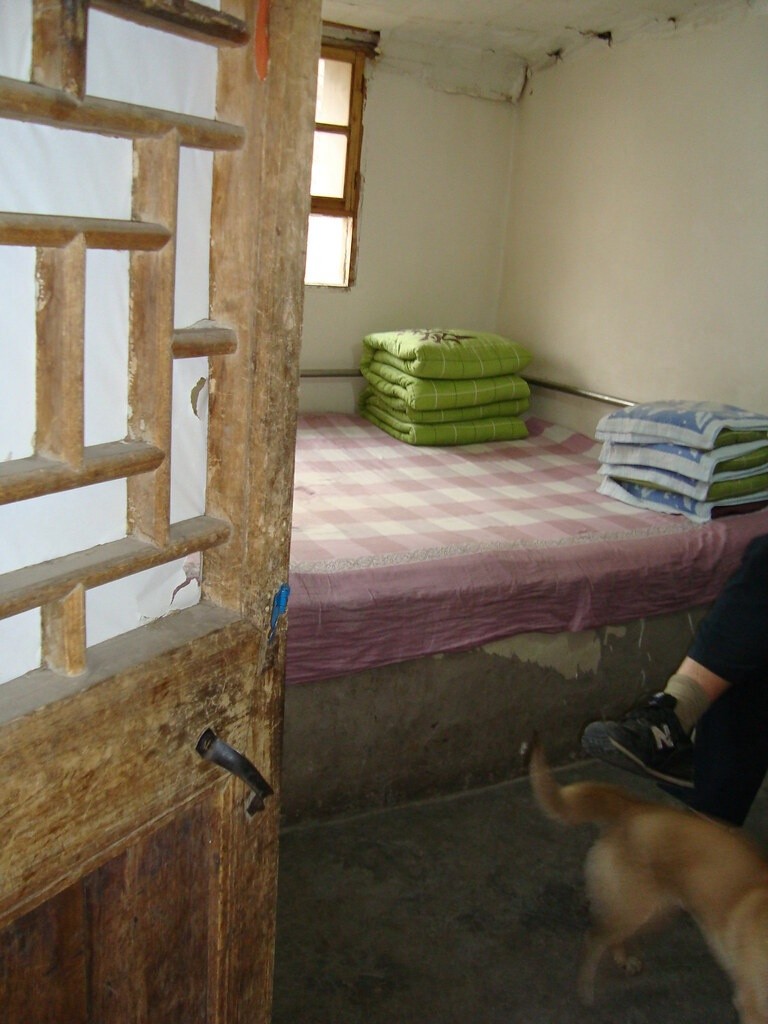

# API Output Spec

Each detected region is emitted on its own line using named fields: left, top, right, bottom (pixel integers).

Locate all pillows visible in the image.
left=594, top=399, right=767, bottom=525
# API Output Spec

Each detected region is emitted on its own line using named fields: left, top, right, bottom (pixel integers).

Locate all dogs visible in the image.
left=530, top=735, right=768, bottom=1024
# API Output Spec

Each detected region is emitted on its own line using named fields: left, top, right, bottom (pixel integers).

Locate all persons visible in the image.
left=579, top=531, right=768, bottom=833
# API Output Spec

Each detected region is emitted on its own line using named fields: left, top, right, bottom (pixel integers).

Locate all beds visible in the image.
left=283, top=408, right=767, bottom=690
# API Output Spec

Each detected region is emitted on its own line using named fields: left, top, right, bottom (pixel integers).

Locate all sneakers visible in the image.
left=582, top=692, right=704, bottom=791
